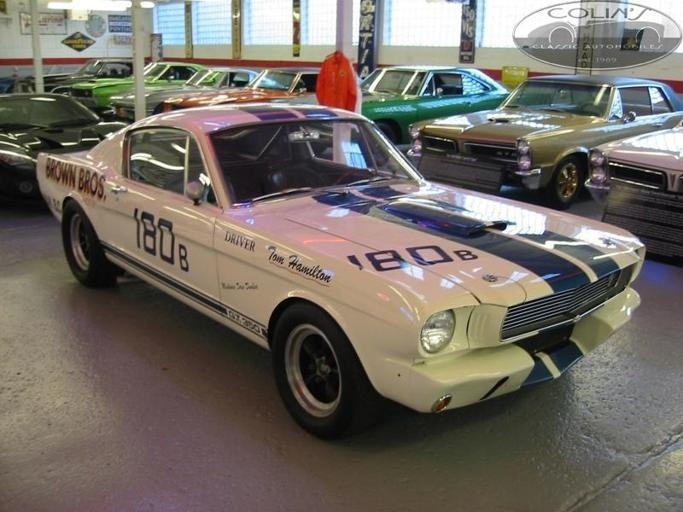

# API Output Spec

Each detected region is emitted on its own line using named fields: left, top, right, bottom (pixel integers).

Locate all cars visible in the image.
left=33, top=99, right=650, bottom=439
